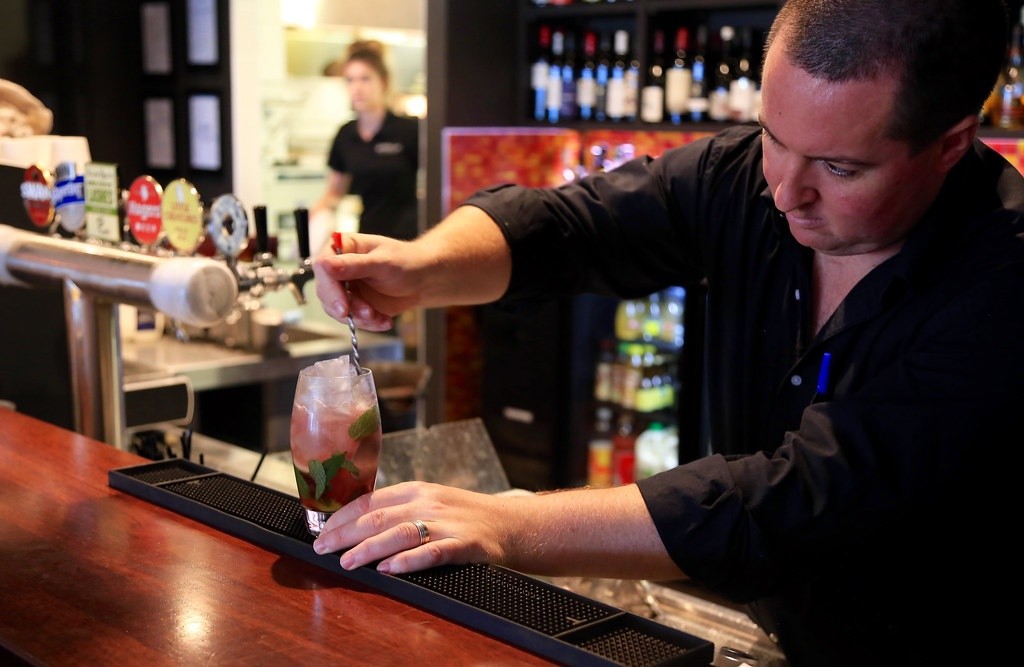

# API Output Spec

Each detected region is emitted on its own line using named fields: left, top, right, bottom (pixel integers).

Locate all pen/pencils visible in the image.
left=816, top=352, right=831, bottom=394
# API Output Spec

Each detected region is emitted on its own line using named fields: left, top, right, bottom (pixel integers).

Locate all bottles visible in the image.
left=990, top=51, right=1024, bottom=132
left=527, top=23, right=764, bottom=186
left=587, top=286, right=685, bottom=489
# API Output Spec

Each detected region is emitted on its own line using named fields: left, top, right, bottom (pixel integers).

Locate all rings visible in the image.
left=412, top=520, right=430, bottom=544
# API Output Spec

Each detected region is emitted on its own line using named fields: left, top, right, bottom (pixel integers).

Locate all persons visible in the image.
left=313, top=0, right=1024, bottom=667
left=310, top=42, right=419, bottom=242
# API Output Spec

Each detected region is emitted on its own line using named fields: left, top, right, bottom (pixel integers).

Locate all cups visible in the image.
left=289, top=364, right=383, bottom=538
